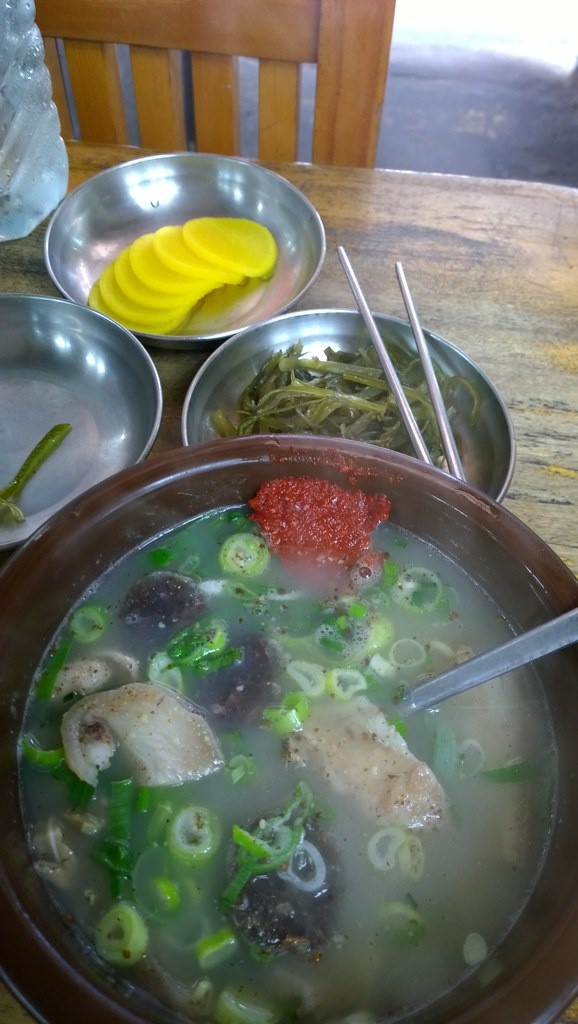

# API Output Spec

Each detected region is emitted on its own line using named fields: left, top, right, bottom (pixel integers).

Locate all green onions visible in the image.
left=19, top=508, right=533, bottom=1024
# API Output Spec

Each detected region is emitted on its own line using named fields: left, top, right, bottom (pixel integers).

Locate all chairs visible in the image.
left=33, top=0, right=396, bottom=169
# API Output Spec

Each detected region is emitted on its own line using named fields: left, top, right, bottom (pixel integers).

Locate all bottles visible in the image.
left=0, top=0, right=69, bottom=241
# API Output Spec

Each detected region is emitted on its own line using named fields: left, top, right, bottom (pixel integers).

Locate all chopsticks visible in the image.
left=335, top=247, right=464, bottom=482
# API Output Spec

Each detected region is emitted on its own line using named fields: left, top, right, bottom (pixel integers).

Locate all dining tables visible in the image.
left=2, top=141, right=577, bottom=579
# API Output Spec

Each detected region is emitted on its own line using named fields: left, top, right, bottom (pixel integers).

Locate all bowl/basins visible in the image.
left=180, top=307, right=517, bottom=506
left=1, top=433, right=578, bottom=1023
left=43, top=152, right=327, bottom=347
left=0, top=291, right=163, bottom=548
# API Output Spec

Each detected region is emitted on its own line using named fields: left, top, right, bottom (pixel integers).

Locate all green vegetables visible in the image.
left=207, top=342, right=481, bottom=474
left=0, top=423, right=72, bottom=520
